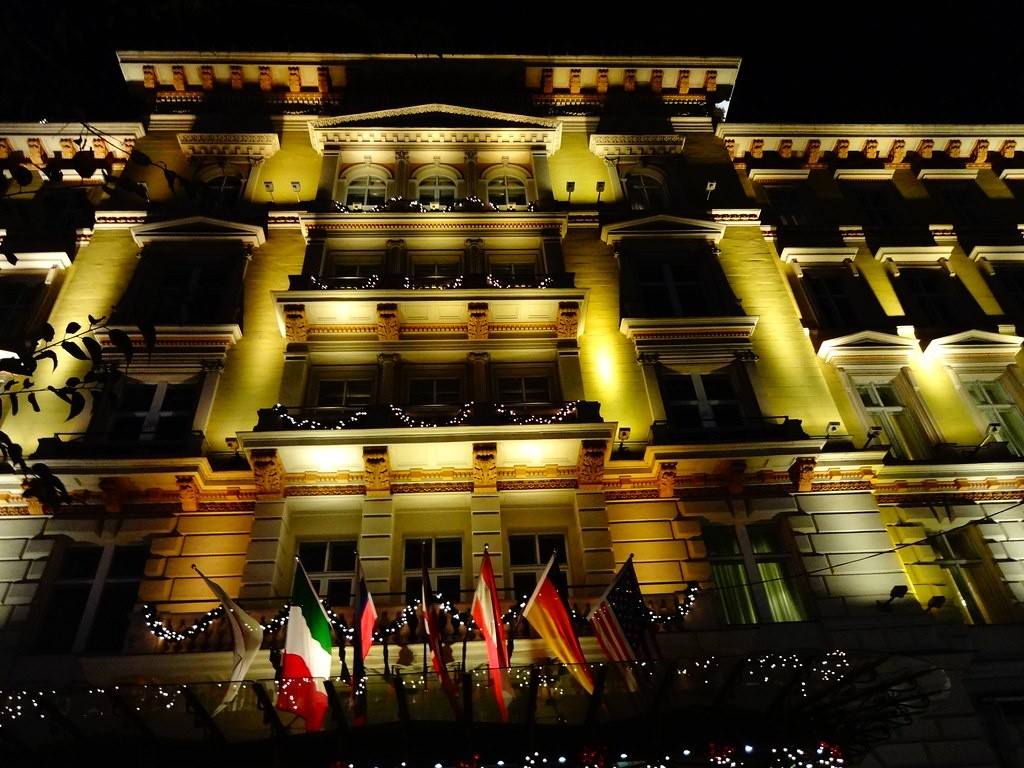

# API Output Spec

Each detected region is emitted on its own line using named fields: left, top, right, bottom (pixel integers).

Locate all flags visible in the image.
left=469, top=549, right=508, bottom=725
left=422, top=545, right=456, bottom=707
left=587, top=557, right=666, bottom=692
left=352, top=557, right=378, bottom=729
left=522, top=554, right=594, bottom=697
left=276, top=560, right=332, bottom=733
left=204, top=578, right=264, bottom=718
